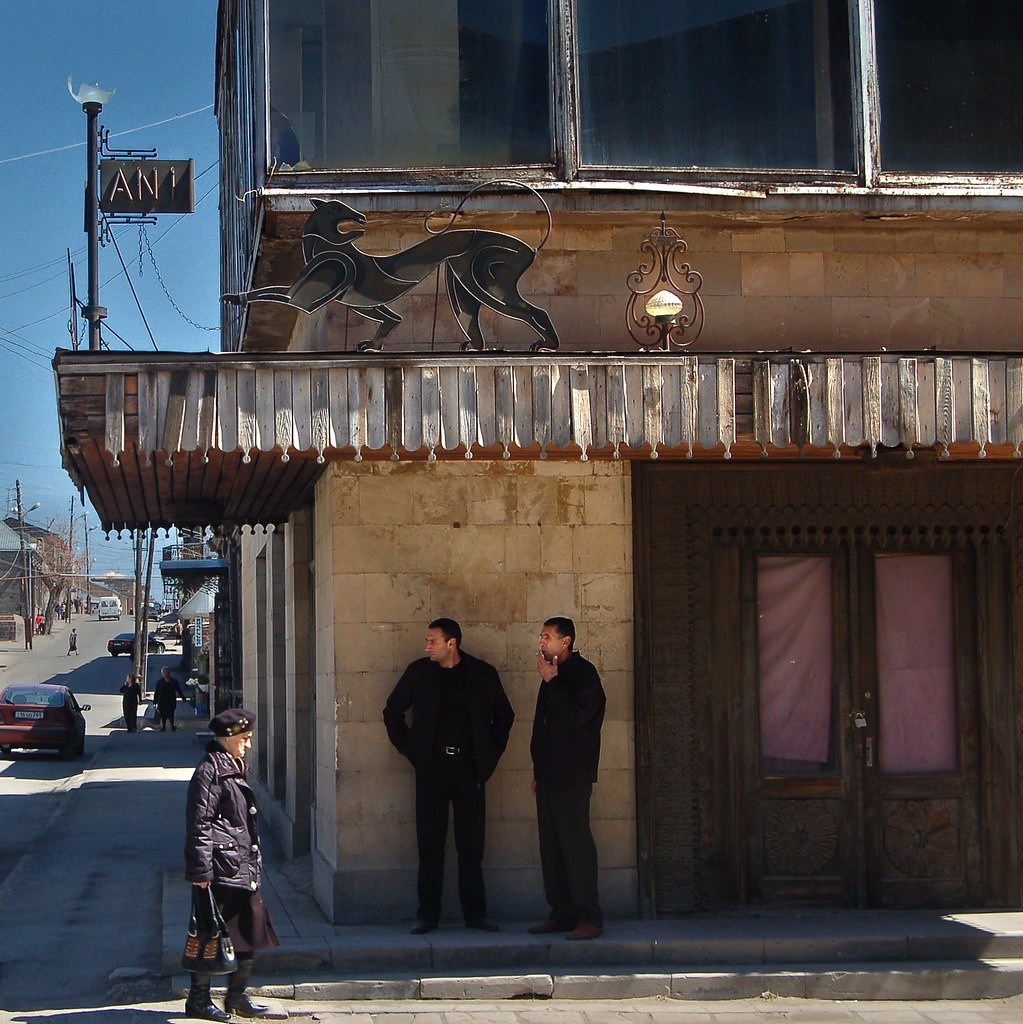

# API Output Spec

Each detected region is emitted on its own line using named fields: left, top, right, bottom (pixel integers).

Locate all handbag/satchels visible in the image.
left=143, top=703, right=161, bottom=725
left=181, top=886, right=238, bottom=975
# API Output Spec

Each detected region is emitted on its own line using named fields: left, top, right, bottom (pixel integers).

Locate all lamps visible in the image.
left=623, top=211, right=707, bottom=356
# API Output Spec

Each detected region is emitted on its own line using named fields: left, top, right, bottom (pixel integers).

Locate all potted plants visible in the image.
left=194, top=654, right=207, bottom=704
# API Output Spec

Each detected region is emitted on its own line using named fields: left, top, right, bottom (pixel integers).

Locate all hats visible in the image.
left=208, top=708, right=256, bottom=737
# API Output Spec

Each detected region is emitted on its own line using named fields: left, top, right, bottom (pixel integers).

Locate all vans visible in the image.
left=98, top=596, right=123, bottom=621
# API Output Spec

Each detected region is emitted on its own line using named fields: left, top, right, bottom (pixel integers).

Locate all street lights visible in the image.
left=65, top=511, right=90, bottom=624
left=85, top=524, right=100, bottom=614
left=18, top=503, right=40, bottom=650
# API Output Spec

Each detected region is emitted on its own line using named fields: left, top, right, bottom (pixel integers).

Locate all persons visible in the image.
left=55, top=602, right=66, bottom=620
left=67, top=628, right=80, bottom=656
left=382, top=618, right=516, bottom=934
left=61, top=599, right=66, bottom=605
left=174, top=619, right=183, bottom=646
left=153, top=666, right=186, bottom=732
left=119, top=673, right=142, bottom=733
left=70, top=600, right=73, bottom=614
left=74, top=596, right=82, bottom=613
left=102, top=601, right=106, bottom=607
left=36, top=613, right=45, bottom=635
left=112, top=602, right=115, bottom=606
left=529, top=616, right=607, bottom=942
left=185, top=708, right=280, bottom=1021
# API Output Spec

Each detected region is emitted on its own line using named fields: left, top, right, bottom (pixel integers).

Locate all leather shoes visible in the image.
left=529, top=920, right=574, bottom=932
left=566, top=923, right=602, bottom=939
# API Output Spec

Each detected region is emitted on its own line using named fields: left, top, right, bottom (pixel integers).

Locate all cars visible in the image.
left=107, top=632, right=165, bottom=657
left=141, top=607, right=158, bottom=622
left=0, top=684, right=91, bottom=760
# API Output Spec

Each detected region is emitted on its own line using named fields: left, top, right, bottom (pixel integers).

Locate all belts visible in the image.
left=434, top=745, right=471, bottom=755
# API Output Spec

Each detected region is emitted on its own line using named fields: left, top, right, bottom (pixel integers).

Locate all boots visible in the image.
left=185, top=972, right=231, bottom=1021
left=225, top=958, right=268, bottom=1013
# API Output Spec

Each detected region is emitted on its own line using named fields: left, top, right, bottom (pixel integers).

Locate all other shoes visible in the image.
left=67, top=654, right=71, bottom=656
left=160, top=727, right=164, bottom=731
left=76, top=653, right=79, bottom=655
left=411, top=920, right=439, bottom=934
left=172, top=726, right=177, bottom=731
left=466, top=919, right=497, bottom=931
